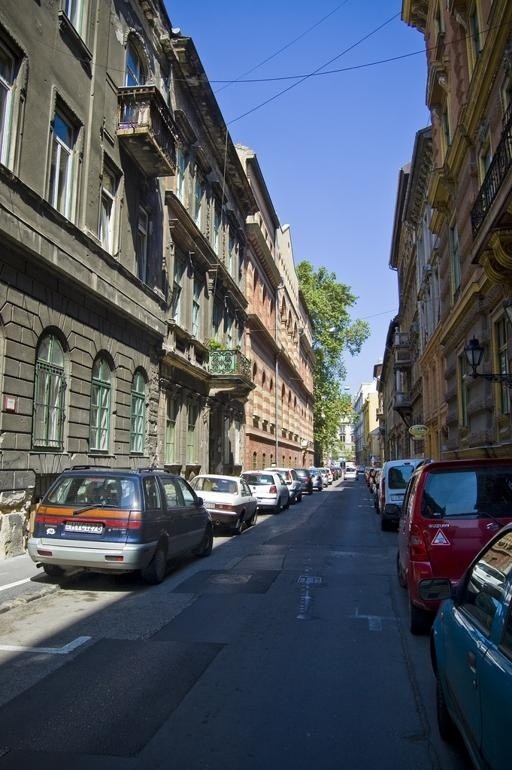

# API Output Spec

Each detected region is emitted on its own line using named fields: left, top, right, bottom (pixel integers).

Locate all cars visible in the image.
left=343, top=465, right=359, bottom=481
left=294, top=466, right=342, bottom=494
left=427, top=521, right=511, bottom=770
left=394, top=457, right=511, bottom=634
left=239, top=470, right=289, bottom=514
left=181, top=474, right=258, bottom=535
left=266, top=466, right=302, bottom=504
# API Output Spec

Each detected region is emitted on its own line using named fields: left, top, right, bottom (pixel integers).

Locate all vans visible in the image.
left=376, top=458, right=434, bottom=531
left=27, top=464, right=214, bottom=583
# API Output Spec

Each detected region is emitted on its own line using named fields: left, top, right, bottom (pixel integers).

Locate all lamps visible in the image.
left=462, top=335, right=511, bottom=390
